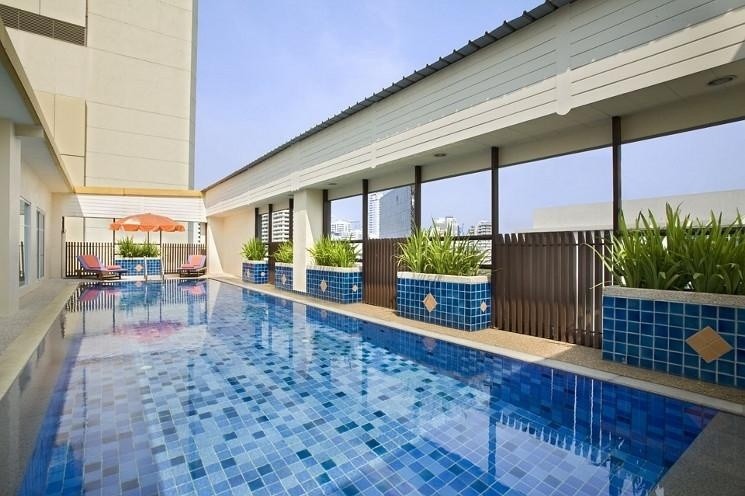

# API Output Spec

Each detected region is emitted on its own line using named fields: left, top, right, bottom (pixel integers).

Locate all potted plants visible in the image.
left=306, top=231, right=366, bottom=306
left=114, top=234, right=163, bottom=277
left=271, top=237, right=294, bottom=292
left=389, top=214, right=492, bottom=332
left=239, top=236, right=269, bottom=285
left=576, top=201, right=745, bottom=392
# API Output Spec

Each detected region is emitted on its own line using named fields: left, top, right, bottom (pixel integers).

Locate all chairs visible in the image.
left=177, top=279, right=208, bottom=297
left=175, top=254, right=207, bottom=278
left=77, top=284, right=128, bottom=303
left=75, top=254, right=127, bottom=282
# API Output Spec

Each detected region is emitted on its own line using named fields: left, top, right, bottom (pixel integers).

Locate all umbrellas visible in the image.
left=113, top=307, right=185, bottom=345
left=109, top=213, right=185, bottom=247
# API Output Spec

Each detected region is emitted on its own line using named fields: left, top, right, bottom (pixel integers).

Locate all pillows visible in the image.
left=181, top=263, right=195, bottom=269
left=105, top=264, right=122, bottom=271
